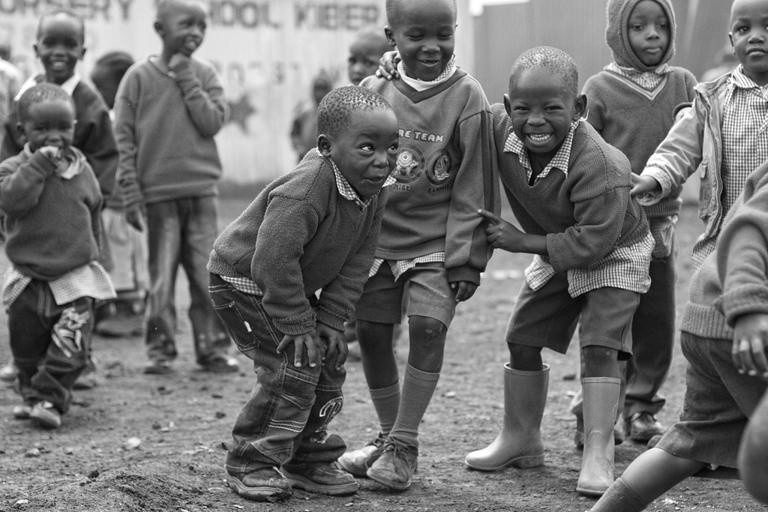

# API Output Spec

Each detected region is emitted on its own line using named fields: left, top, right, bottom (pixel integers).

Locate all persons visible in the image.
left=572, top=0, right=701, bottom=449
left=206, top=85, right=400, bottom=502
left=339, top=0, right=502, bottom=489
left=1, top=83, right=104, bottom=428
left=627, top=0, right=767, bottom=262
left=1, top=12, right=120, bottom=204
left=342, top=27, right=402, bottom=361
left=376, top=50, right=656, bottom=497
left=588, top=160, right=768, bottom=512
left=90, top=50, right=154, bottom=307
left=290, top=75, right=334, bottom=164
left=111, top=0, right=242, bottom=377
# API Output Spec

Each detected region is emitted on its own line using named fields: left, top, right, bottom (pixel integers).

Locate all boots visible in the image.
left=465, top=363, right=550, bottom=471
left=576, top=377, right=620, bottom=495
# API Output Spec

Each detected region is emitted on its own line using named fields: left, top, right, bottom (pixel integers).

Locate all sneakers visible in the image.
left=73, top=360, right=96, bottom=388
left=573, top=416, right=622, bottom=445
left=196, top=350, right=238, bottom=371
left=146, top=358, right=169, bottom=373
left=337, top=431, right=385, bottom=477
left=622, top=410, right=665, bottom=441
left=280, top=461, right=358, bottom=495
left=366, top=437, right=419, bottom=490
left=95, top=300, right=144, bottom=334
left=30, top=400, right=61, bottom=426
left=225, top=467, right=289, bottom=499
left=13, top=400, right=32, bottom=416
